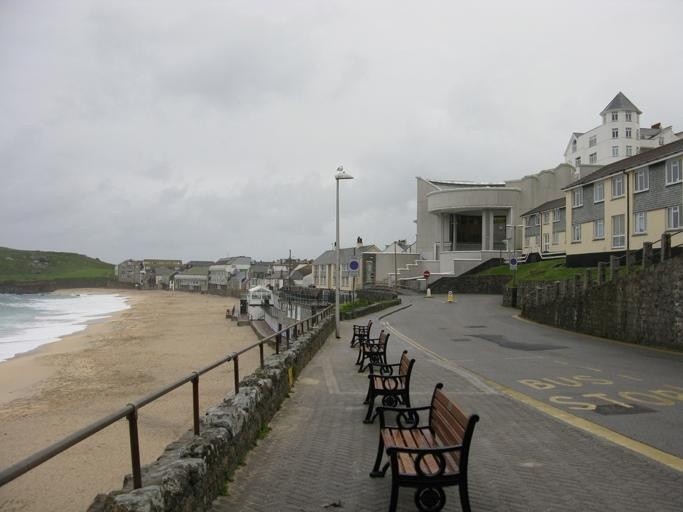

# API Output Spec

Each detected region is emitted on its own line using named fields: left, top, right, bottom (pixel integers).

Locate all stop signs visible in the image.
left=423, top=271, right=429, bottom=279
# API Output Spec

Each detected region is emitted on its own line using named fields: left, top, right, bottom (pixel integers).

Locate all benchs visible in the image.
left=367, top=381, right=479, bottom=512
left=350, top=319, right=373, bottom=347
left=362, top=348, right=416, bottom=421
left=354, top=328, right=389, bottom=373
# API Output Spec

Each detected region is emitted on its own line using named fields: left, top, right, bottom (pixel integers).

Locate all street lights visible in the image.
left=384, top=239, right=407, bottom=294
left=506, top=224, right=524, bottom=252
left=332, top=164, right=353, bottom=338
